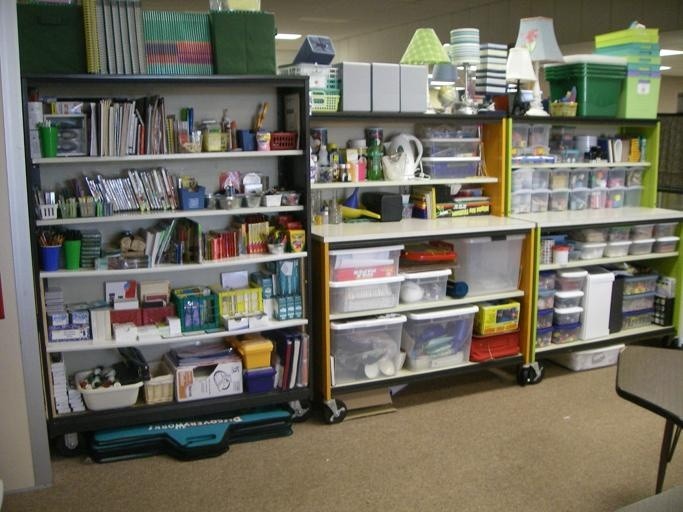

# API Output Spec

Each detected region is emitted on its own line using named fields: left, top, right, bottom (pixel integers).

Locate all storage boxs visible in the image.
left=165, top=334, right=276, bottom=404
left=544, top=27, right=663, bottom=120
left=333, top=62, right=429, bottom=113
left=72, top=366, right=143, bottom=411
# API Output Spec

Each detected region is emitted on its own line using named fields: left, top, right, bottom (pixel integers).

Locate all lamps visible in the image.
left=474, top=41, right=509, bottom=97
left=447, top=27, right=480, bottom=113
left=431, top=43, right=461, bottom=114
left=513, top=15, right=564, bottom=117
left=400, top=27, right=449, bottom=65
left=503, top=48, right=534, bottom=116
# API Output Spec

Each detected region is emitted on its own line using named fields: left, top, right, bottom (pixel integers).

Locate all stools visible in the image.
left=618, top=342, right=682, bottom=495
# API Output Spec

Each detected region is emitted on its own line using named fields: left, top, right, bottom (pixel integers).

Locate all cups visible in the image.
left=39, top=245, right=62, bottom=272
left=38, top=127, right=58, bottom=159
left=540, top=236, right=554, bottom=263
left=64, top=240, right=81, bottom=271
left=176, top=135, right=202, bottom=153
left=401, top=203, right=414, bottom=222
left=266, top=243, right=284, bottom=254
left=381, top=152, right=406, bottom=181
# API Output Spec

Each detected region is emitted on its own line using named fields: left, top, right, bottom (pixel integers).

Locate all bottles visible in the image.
left=575, top=133, right=648, bottom=163
left=310, top=126, right=382, bottom=184
left=199, top=118, right=270, bottom=152
left=80, top=365, right=121, bottom=390
left=447, top=279, right=468, bottom=298
left=279, top=187, right=300, bottom=205
left=552, top=246, right=570, bottom=264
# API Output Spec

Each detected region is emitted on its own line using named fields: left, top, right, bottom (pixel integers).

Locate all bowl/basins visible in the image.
left=204, top=194, right=261, bottom=209
left=310, top=191, right=343, bottom=226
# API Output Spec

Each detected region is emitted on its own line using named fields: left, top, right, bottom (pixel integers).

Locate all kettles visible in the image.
left=382, top=133, right=427, bottom=182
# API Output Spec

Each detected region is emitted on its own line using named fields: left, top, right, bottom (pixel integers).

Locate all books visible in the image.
left=66, top=95, right=167, bottom=157
left=76, top=166, right=176, bottom=213
left=83, top=1, right=216, bottom=78
left=475, top=41, right=509, bottom=98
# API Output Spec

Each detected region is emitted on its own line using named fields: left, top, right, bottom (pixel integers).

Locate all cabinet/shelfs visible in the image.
left=19, top=70, right=313, bottom=459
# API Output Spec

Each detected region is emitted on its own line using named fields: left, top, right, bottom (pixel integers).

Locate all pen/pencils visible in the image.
left=38, top=231, right=65, bottom=247
left=60, top=228, right=83, bottom=240
left=255, top=102, right=269, bottom=129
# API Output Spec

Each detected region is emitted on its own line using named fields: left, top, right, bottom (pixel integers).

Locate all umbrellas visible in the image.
left=144, top=212, right=269, bottom=265
